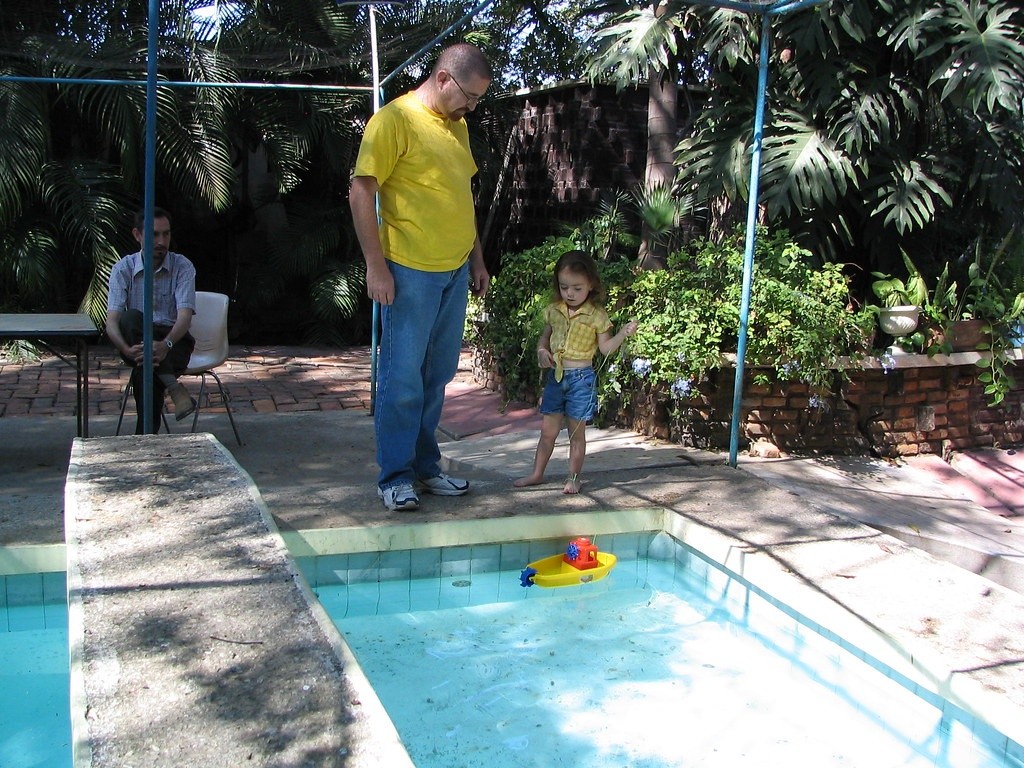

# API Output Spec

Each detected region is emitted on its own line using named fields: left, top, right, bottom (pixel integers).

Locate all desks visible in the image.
left=0, top=314, right=97, bottom=438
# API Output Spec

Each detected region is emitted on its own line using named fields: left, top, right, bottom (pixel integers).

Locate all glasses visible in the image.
left=448, top=73, right=483, bottom=104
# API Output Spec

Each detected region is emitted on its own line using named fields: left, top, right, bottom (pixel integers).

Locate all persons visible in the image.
left=348, top=44, right=496, bottom=511
left=106, top=206, right=197, bottom=435
left=512, top=250, right=638, bottom=495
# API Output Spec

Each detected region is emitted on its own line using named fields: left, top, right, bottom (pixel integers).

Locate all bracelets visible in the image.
left=538, top=349, right=545, bottom=352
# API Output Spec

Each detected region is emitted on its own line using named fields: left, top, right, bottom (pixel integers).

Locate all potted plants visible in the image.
left=898, top=220, right=1024, bottom=415
left=980, top=268, right=1024, bottom=348
left=870, top=269, right=925, bottom=355
left=835, top=300, right=876, bottom=356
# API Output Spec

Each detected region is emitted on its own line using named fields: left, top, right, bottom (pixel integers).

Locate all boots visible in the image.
left=168, top=383, right=197, bottom=421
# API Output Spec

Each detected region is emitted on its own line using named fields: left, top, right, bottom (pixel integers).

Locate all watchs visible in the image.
left=163, top=337, right=173, bottom=352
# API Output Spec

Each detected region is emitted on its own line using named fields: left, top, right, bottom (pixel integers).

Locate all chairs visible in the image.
left=116, top=291, right=242, bottom=445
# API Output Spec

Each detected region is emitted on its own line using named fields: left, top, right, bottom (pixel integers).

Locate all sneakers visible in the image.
left=377, top=482, right=419, bottom=511
left=413, top=474, right=469, bottom=495
